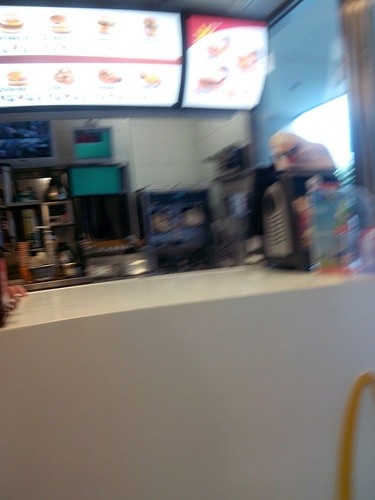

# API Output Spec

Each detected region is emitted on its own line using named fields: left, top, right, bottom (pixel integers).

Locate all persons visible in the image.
left=0, top=242, right=24, bottom=312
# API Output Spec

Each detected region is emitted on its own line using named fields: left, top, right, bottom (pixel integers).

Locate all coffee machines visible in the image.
left=261, top=167, right=338, bottom=272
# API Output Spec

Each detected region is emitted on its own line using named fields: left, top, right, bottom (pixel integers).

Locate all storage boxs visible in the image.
left=16, top=242, right=32, bottom=283
left=15, top=177, right=53, bottom=202
